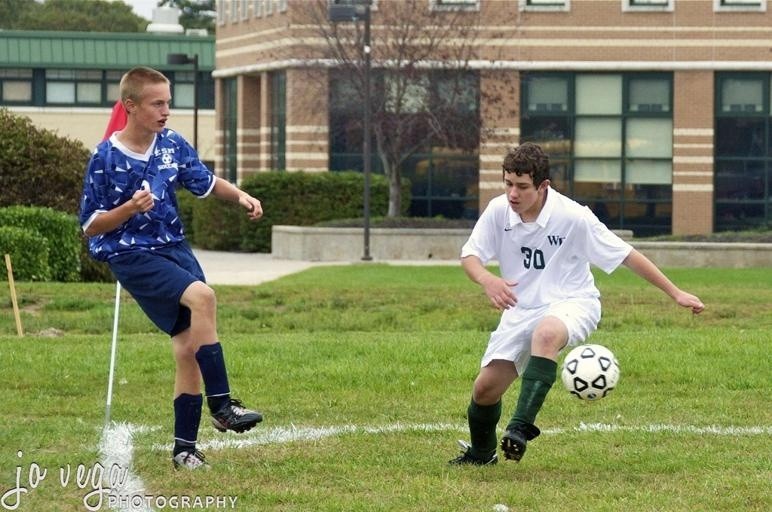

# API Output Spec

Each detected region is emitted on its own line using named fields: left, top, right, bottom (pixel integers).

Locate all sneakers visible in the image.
left=210, top=403, right=263, bottom=433
left=173, top=449, right=209, bottom=471
left=446, top=449, right=499, bottom=465
left=500, top=422, right=540, bottom=461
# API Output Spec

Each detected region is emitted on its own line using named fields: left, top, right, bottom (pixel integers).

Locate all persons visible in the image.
left=446, top=141, right=707, bottom=467
left=78, top=64, right=264, bottom=474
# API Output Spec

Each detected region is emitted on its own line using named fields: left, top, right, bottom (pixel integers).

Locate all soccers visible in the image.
left=560, top=343, right=620, bottom=402
